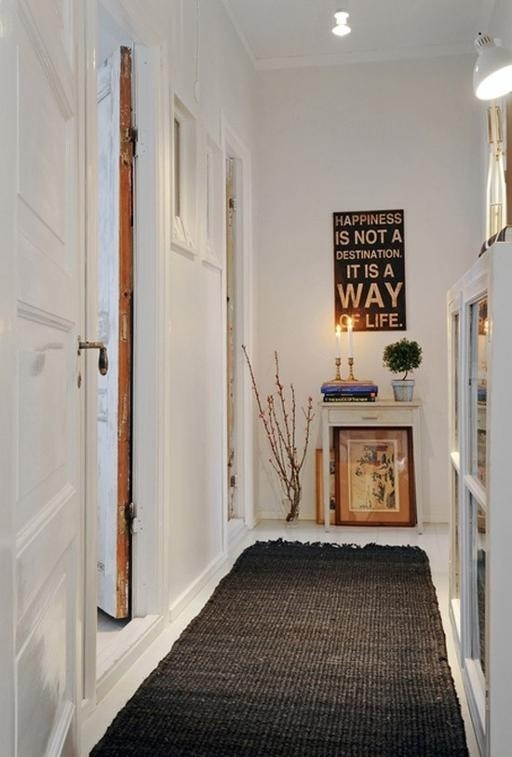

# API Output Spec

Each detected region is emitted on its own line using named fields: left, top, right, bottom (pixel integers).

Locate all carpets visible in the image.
left=88, top=541, right=470, bottom=756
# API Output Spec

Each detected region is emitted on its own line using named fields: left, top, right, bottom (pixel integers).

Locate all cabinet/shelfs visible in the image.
left=445, top=241, right=512, bottom=756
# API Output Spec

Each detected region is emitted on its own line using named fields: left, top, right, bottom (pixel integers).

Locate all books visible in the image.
left=321, top=383, right=378, bottom=403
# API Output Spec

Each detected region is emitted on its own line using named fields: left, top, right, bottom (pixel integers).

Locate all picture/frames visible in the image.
left=332, top=425, right=418, bottom=528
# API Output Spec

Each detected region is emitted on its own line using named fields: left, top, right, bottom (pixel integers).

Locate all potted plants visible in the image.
left=383, top=340, right=422, bottom=403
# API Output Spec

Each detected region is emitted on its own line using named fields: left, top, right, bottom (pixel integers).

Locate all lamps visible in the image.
left=470, top=30, right=512, bottom=237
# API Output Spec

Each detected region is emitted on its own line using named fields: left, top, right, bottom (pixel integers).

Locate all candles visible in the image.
left=332, top=316, right=357, bottom=358
